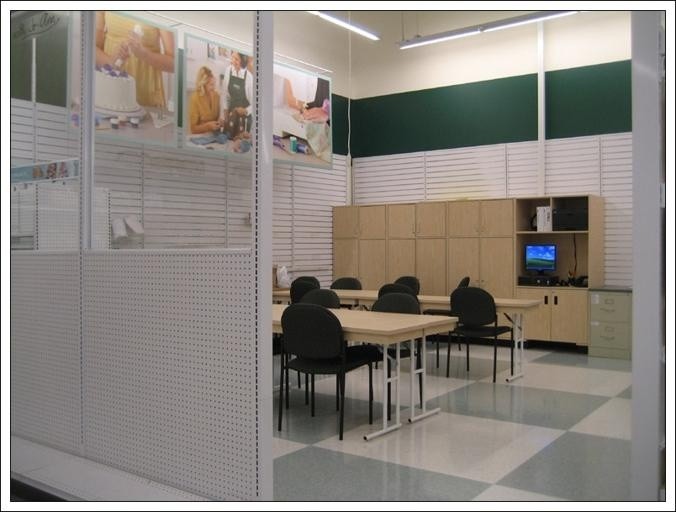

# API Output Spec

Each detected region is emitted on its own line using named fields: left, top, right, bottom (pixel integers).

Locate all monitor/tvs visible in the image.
left=523, top=241, right=557, bottom=276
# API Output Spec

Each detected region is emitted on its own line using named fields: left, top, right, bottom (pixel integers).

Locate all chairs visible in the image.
left=378, top=283, right=419, bottom=301
left=278, top=302, right=375, bottom=438
left=331, top=275, right=365, bottom=294
left=393, top=276, right=421, bottom=296
left=367, top=292, right=422, bottom=421
left=423, top=275, right=470, bottom=370
left=290, top=276, right=320, bottom=311
left=447, top=285, right=514, bottom=382
left=300, top=288, right=342, bottom=312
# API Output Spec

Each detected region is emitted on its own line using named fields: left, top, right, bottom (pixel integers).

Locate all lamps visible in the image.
left=394, top=10, right=571, bottom=52
left=311, top=12, right=382, bottom=42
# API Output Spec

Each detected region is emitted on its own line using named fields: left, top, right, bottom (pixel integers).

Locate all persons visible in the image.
left=300, top=75, right=330, bottom=131
left=93, top=10, right=177, bottom=109
left=186, top=66, right=224, bottom=135
left=223, top=50, right=253, bottom=140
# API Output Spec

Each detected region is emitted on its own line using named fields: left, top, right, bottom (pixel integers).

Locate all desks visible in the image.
left=271, top=303, right=460, bottom=442
left=274, top=287, right=543, bottom=383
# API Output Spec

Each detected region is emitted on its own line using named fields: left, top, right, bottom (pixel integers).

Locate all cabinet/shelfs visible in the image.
left=589, top=289, right=634, bottom=376
left=514, top=194, right=608, bottom=351
left=332, top=206, right=387, bottom=310
left=445, top=201, right=517, bottom=342
left=386, top=202, right=449, bottom=315
